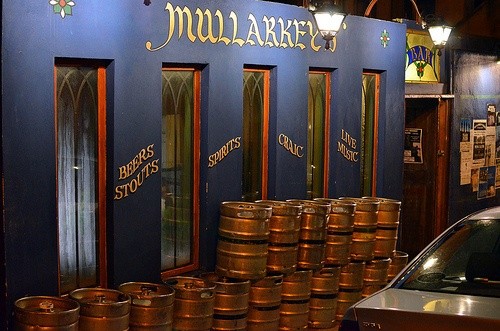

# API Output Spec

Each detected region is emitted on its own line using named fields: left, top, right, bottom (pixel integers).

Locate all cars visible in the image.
left=337, top=206, right=500, bottom=330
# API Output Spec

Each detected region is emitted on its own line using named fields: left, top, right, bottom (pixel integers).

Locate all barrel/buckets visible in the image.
left=215, top=201, right=272, bottom=280
left=335, top=261, right=365, bottom=321
left=199, top=272, right=250, bottom=331
left=308, top=268, right=340, bottom=330
left=279, top=268, right=313, bottom=331
left=12, top=295, right=80, bottom=331
left=362, top=196, right=402, bottom=257
left=313, top=198, right=356, bottom=265
left=162, top=276, right=216, bottom=331
left=387, top=251, right=409, bottom=283
left=339, top=196, right=380, bottom=261
left=286, top=199, right=331, bottom=269
left=362, top=257, right=392, bottom=299
left=254, top=200, right=303, bottom=273
left=68, top=288, right=131, bottom=331
left=247, top=273, right=283, bottom=331
left=118, top=282, right=175, bottom=331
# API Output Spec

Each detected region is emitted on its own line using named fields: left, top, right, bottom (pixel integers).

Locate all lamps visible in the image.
left=423, top=14, right=456, bottom=58
left=308, top=0, right=349, bottom=50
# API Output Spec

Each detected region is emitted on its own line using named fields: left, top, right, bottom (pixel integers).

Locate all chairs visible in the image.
left=464, top=252, right=500, bottom=281
